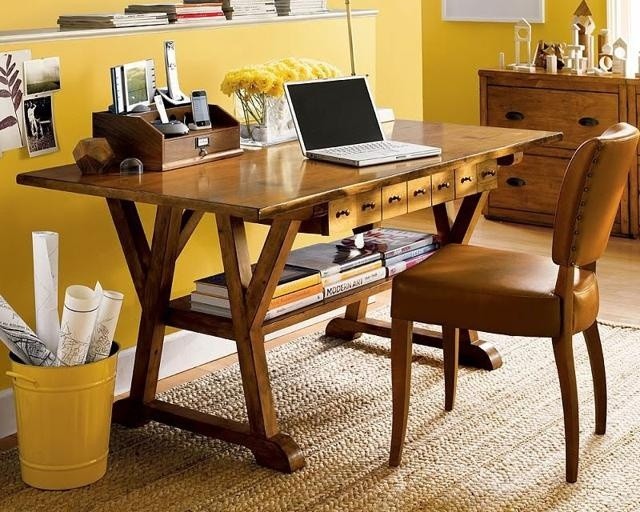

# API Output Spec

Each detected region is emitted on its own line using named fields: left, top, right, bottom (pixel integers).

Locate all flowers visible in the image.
left=220, top=55, right=342, bottom=141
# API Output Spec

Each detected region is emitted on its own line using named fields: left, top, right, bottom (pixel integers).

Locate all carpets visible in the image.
left=0, top=298, right=640, bottom=512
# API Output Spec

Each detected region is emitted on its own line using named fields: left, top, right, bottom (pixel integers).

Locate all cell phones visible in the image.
left=191, top=89, right=210, bottom=127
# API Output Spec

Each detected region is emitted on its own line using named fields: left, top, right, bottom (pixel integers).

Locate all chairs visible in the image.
left=389, top=121, right=640, bottom=482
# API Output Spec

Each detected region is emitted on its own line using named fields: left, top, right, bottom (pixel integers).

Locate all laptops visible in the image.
left=283, top=75, right=441, bottom=167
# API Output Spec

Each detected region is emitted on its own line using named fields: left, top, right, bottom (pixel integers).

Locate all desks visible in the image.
left=15, top=117, right=565, bottom=475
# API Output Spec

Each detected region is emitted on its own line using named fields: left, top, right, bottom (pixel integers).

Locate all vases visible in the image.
left=234, top=89, right=298, bottom=143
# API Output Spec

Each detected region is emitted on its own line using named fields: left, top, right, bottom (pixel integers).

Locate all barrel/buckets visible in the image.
left=7, top=341, right=121, bottom=491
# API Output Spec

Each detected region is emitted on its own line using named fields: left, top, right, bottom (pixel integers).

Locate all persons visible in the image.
left=27, top=102, right=41, bottom=137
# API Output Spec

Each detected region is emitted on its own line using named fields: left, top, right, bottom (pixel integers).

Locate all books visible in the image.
left=57, top=0, right=328, bottom=30
left=190, top=226, right=440, bottom=323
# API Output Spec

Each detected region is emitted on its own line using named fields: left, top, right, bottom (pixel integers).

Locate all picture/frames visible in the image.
left=440, top=0, right=546, bottom=24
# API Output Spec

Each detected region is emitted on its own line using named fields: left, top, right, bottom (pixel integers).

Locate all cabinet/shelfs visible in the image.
left=478, top=68, right=640, bottom=238
